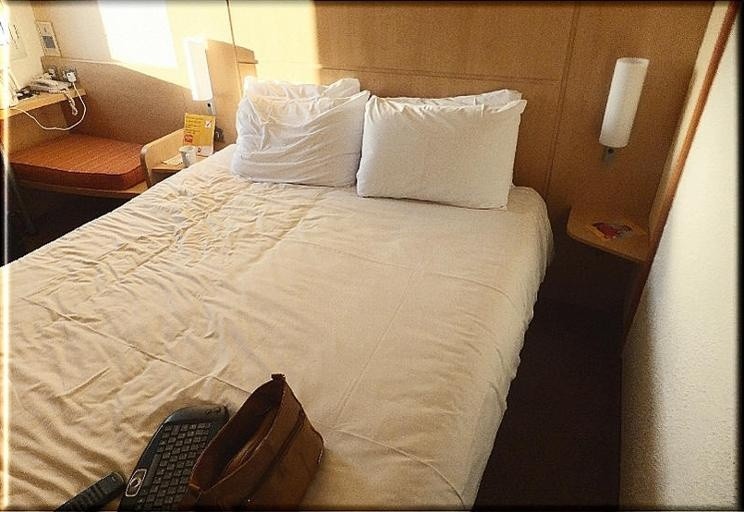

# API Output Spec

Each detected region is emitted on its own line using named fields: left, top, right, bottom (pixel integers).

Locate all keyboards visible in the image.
left=117, top=405, right=227, bottom=511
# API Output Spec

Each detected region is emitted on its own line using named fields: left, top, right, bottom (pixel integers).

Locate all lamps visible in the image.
left=599, top=57, right=652, bottom=151
left=181, top=34, right=217, bottom=114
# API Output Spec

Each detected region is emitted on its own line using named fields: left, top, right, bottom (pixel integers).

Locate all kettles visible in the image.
left=0, top=67, right=20, bottom=111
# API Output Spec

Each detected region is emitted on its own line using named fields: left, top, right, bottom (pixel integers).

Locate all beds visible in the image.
left=0, top=141, right=553, bottom=509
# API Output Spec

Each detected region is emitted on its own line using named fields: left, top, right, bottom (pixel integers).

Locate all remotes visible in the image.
left=51, top=472, right=128, bottom=510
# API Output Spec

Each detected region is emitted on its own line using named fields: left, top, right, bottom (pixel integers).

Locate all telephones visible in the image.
left=30, top=79, right=71, bottom=92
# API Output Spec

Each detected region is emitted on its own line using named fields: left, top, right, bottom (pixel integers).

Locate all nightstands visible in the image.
left=138, top=128, right=223, bottom=189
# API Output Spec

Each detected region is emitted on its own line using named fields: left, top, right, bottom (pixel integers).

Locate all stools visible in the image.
left=9, top=135, right=150, bottom=199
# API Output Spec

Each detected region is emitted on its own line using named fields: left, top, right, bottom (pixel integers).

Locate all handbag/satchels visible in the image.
left=180, top=374, right=324, bottom=509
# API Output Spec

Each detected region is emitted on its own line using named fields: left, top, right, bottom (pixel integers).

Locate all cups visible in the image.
left=179, top=145, right=195, bottom=167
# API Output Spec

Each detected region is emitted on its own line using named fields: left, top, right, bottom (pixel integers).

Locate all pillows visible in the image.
left=242, top=75, right=361, bottom=101
left=355, top=95, right=526, bottom=210
left=383, top=88, right=523, bottom=109
left=231, top=90, right=373, bottom=188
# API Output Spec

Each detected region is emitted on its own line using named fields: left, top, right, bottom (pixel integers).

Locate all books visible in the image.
left=182, top=113, right=216, bottom=157
